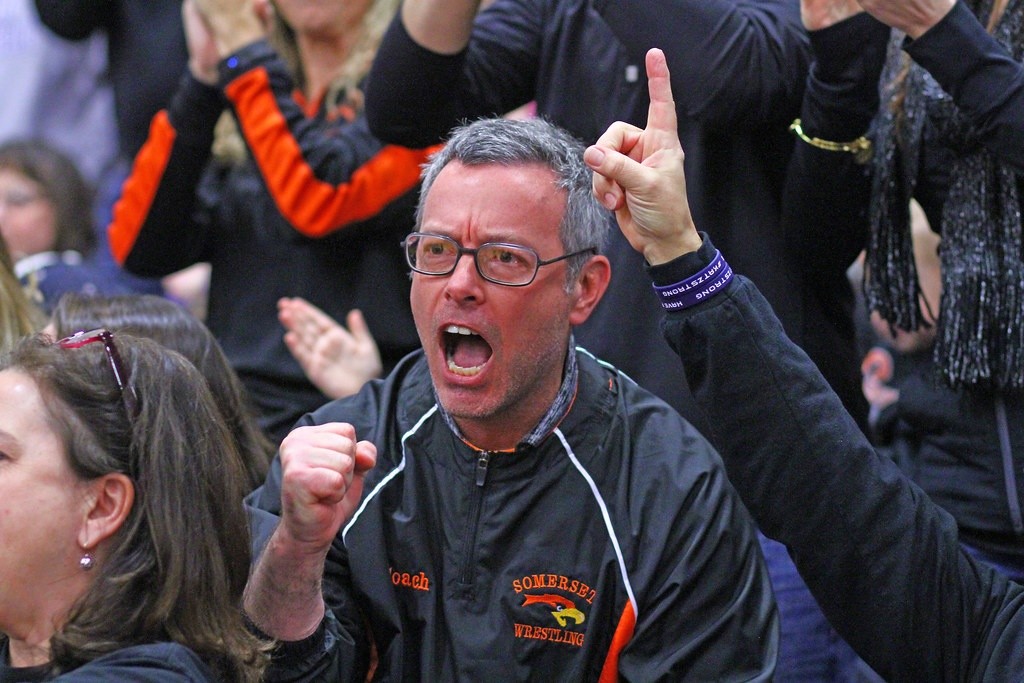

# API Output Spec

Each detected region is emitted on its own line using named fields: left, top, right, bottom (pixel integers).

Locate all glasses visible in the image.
left=55, top=328, right=143, bottom=458
left=400, top=232, right=598, bottom=286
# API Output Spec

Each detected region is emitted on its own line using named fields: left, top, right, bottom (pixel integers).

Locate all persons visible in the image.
left=0, top=0, right=1024, bottom=683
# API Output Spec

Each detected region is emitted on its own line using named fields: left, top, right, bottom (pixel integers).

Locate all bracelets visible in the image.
left=788, top=118, right=874, bottom=153
left=652, top=247, right=737, bottom=313
